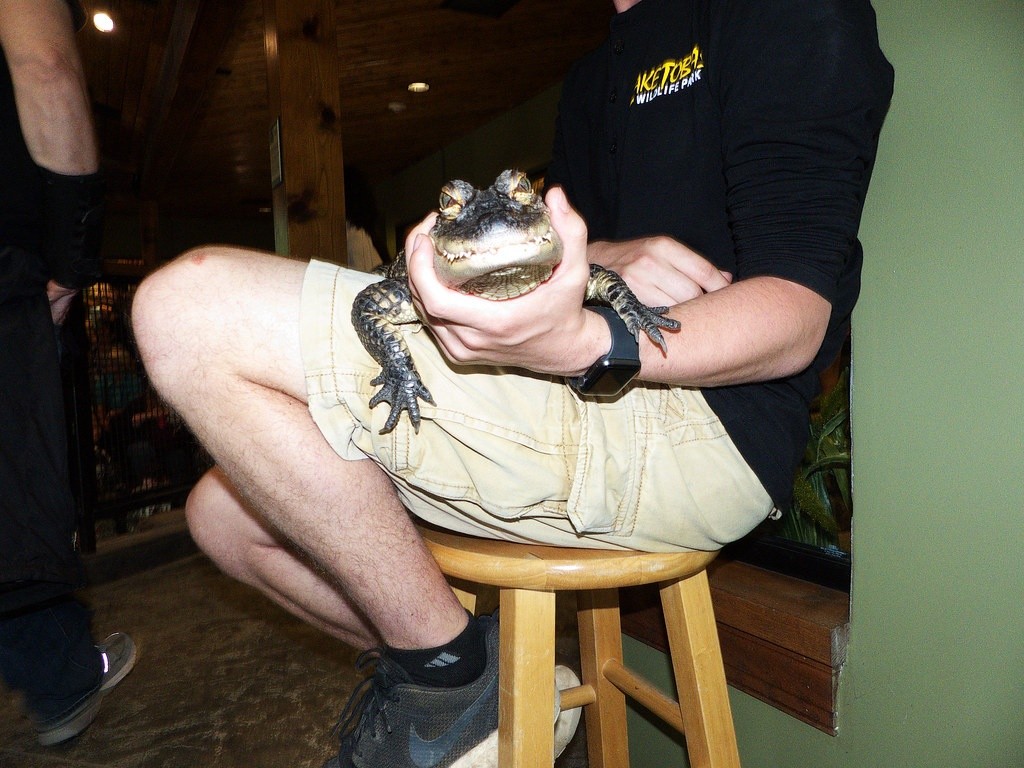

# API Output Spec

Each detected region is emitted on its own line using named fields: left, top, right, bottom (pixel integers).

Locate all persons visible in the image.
left=0, top=1, right=138, bottom=750
left=126, top=2, right=897, bottom=768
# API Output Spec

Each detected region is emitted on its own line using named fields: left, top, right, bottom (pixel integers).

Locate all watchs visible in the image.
left=566, top=306, right=641, bottom=401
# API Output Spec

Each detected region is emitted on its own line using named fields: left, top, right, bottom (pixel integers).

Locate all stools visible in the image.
left=409, top=521, right=740, bottom=768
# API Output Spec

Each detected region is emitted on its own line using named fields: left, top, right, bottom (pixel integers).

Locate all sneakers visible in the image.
left=320, top=612, right=582, bottom=768
left=34, top=629, right=137, bottom=746
